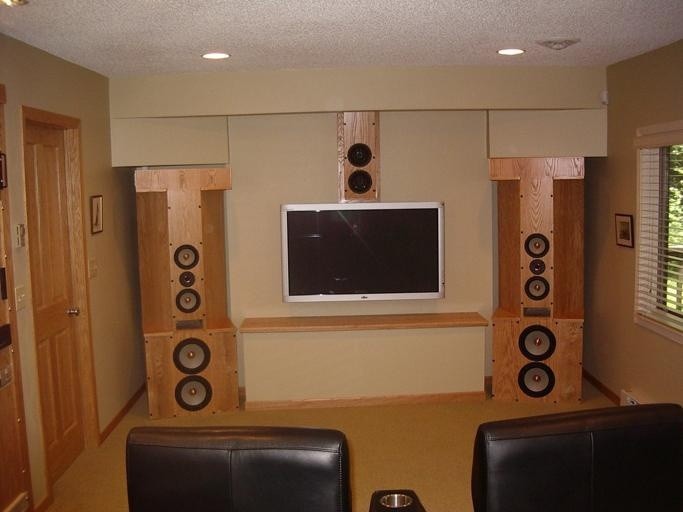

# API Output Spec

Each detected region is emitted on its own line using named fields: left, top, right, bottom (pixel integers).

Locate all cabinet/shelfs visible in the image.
left=240, top=312, right=490, bottom=412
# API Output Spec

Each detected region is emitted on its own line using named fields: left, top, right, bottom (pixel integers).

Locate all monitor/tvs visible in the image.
left=279, top=201, right=445, bottom=304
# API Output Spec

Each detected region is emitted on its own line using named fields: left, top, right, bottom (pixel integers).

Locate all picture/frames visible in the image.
left=90, top=195, right=103, bottom=234
left=615, top=213, right=634, bottom=248
left=0, top=153, right=8, bottom=189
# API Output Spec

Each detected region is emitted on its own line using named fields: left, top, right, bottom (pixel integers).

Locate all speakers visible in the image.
left=142, top=332, right=241, bottom=421
left=519, top=178, right=556, bottom=320
left=337, top=111, right=382, bottom=204
left=166, top=189, right=207, bottom=330
left=491, top=319, right=584, bottom=404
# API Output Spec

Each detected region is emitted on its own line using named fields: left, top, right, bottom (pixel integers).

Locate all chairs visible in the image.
left=125, top=426, right=351, bottom=512
left=471, top=403, right=683, bottom=512
